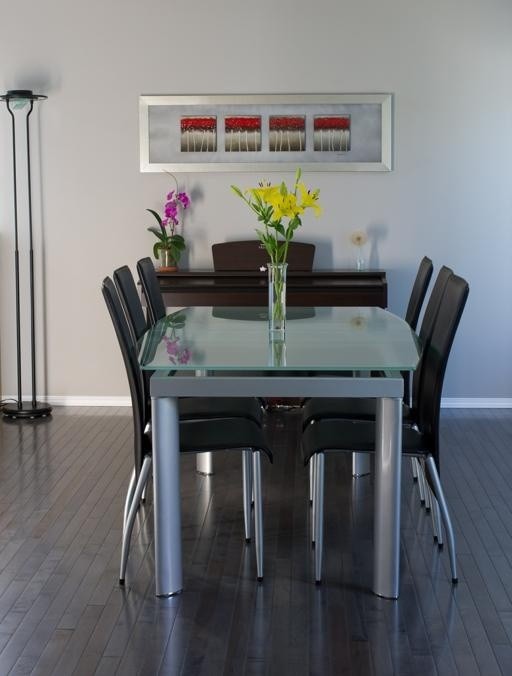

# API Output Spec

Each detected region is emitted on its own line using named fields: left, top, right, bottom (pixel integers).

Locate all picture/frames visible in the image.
left=139, top=90, right=392, bottom=176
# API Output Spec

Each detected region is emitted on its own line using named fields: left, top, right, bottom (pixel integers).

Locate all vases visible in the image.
left=159, top=248, right=177, bottom=272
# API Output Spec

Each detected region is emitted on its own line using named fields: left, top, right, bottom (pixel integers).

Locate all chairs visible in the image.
left=300, top=254, right=471, bottom=585
left=102, top=255, right=275, bottom=590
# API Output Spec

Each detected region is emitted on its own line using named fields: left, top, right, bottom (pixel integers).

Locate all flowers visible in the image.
left=145, top=166, right=192, bottom=262
left=350, top=229, right=367, bottom=247
left=229, top=166, right=326, bottom=320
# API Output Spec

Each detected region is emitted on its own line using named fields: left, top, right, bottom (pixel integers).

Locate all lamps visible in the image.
left=0, top=89, right=56, bottom=421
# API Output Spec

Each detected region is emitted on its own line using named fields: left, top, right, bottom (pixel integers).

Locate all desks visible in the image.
left=136, top=266, right=390, bottom=310
left=140, top=303, right=422, bottom=598
